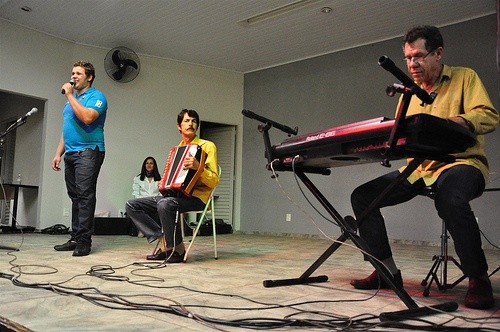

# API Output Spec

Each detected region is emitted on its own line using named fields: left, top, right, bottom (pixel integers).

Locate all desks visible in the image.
left=0, top=183, right=39, bottom=232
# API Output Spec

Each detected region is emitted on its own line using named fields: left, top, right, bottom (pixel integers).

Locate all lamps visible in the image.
left=248, top=0, right=324, bottom=25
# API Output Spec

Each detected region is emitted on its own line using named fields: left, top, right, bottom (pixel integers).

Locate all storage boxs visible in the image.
left=92, top=217, right=131, bottom=235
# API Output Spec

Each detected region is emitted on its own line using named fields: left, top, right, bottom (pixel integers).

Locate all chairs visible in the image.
left=152, top=164, right=222, bottom=263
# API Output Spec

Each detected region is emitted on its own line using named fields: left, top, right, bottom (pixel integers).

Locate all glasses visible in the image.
left=403, top=47, right=436, bottom=64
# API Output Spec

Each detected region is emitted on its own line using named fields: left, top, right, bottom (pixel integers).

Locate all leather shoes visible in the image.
left=164, top=249, right=188, bottom=263
left=146, top=248, right=167, bottom=260
left=350, top=267, right=404, bottom=291
left=463, top=275, right=496, bottom=309
left=72, top=246, right=91, bottom=257
left=52, top=239, right=77, bottom=251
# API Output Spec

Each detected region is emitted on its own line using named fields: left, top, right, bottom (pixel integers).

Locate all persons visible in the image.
left=132, top=156, right=162, bottom=237
left=125, top=108, right=219, bottom=263
left=349, top=24, right=500, bottom=309
left=52, top=61, right=108, bottom=256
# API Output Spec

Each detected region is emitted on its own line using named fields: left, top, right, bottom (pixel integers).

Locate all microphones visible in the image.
left=62, top=79, right=76, bottom=94
left=15, top=107, right=38, bottom=124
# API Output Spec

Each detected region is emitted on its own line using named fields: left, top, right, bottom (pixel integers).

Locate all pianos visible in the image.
left=263, top=112, right=479, bottom=322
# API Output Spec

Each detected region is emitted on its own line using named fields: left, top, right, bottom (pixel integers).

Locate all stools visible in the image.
left=419, top=186, right=467, bottom=295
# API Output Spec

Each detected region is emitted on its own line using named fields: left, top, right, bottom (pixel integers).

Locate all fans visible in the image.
left=104, top=46, right=140, bottom=83
